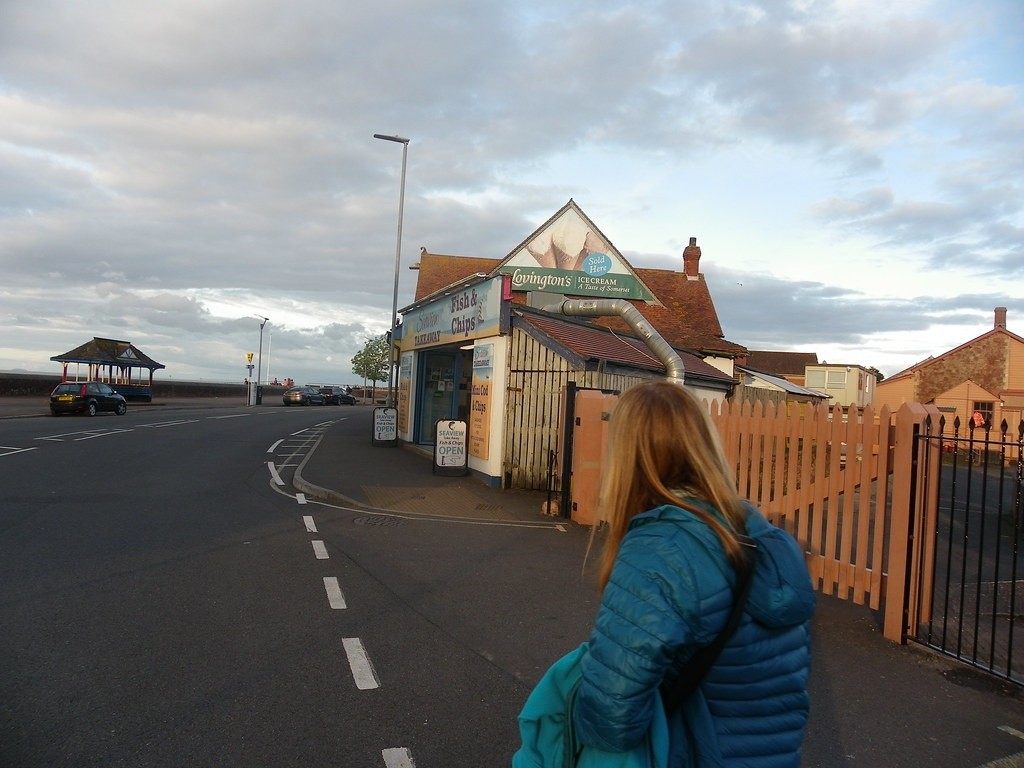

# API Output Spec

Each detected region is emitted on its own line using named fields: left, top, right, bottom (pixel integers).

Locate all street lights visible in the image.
left=372, top=132, right=411, bottom=409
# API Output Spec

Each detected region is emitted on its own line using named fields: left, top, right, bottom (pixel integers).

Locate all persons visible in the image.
left=570, top=377, right=814, bottom=768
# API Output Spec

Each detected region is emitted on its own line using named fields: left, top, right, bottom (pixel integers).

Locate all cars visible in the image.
left=48, top=379, right=128, bottom=416
left=282, top=385, right=327, bottom=407
left=316, top=386, right=356, bottom=406
